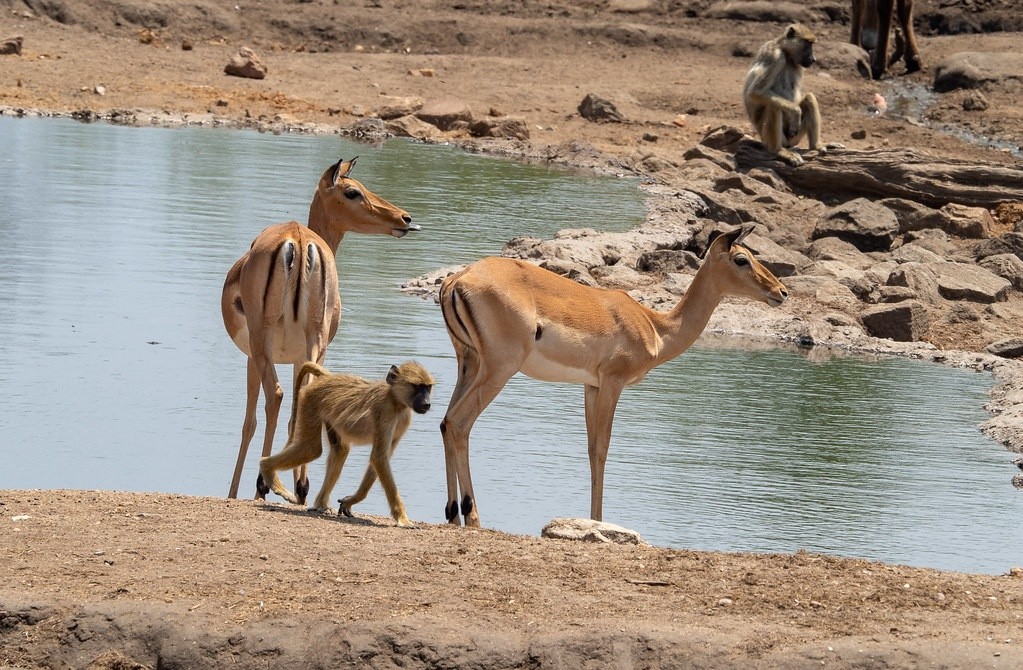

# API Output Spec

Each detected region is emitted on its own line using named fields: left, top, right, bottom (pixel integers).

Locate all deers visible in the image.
left=438, top=224, right=788, bottom=532
left=220, top=154, right=421, bottom=505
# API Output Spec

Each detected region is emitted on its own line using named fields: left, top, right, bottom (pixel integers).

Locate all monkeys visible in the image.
left=256, top=361, right=434, bottom=529
left=741, top=23, right=828, bottom=166
left=851, top=0, right=922, bottom=80
